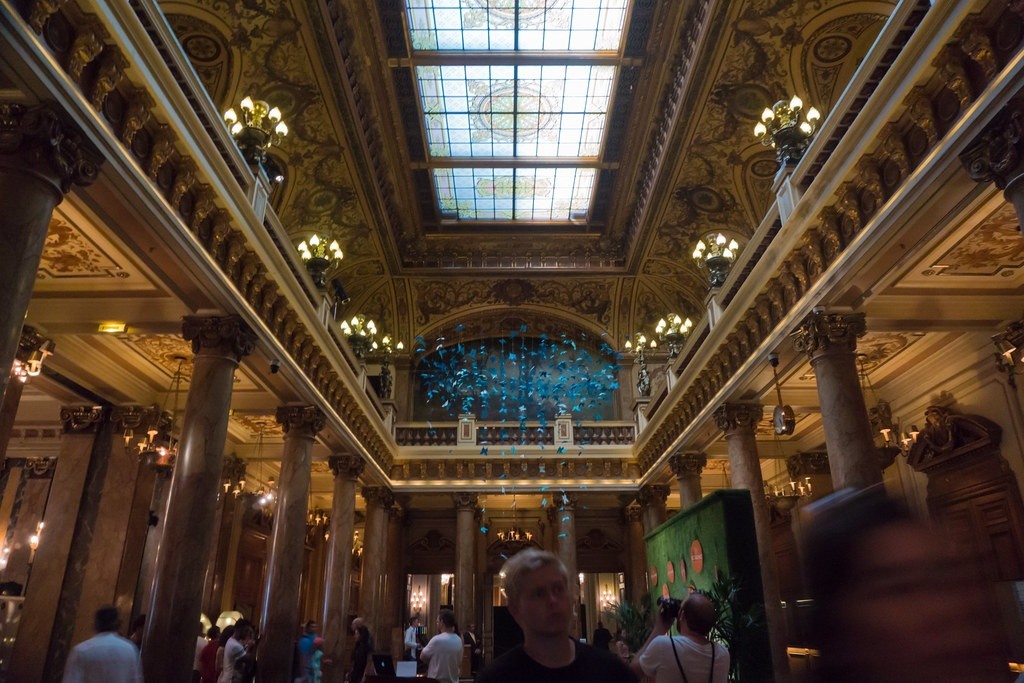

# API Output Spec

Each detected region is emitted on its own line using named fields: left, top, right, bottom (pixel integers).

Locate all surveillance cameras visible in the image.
left=768, top=354, right=779, bottom=367
left=813, top=306, right=825, bottom=315
left=270, top=360, right=281, bottom=374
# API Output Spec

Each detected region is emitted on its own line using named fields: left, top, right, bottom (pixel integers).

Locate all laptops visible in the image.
left=372, top=654, right=396, bottom=678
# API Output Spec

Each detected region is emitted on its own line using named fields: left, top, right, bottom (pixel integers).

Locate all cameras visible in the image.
left=657, top=598, right=683, bottom=622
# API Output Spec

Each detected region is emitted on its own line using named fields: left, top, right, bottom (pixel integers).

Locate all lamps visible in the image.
left=13, top=326, right=56, bottom=383
left=268, top=162, right=285, bottom=184
left=224, top=96, right=288, bottom=165
left=222, top=423, right=278, bottom=511
left=367, top=334, right=404, bottom=397
left=297, top=233, right=344, bottom=289
left=991, top=322, right=1024, bottom=388
left=123, top=357, right=188, bottom=475
left=691, top=230, right=738, bottom=290
left=855, top=354, right=919, bottom=473
left=754, top=95, right=822, bottom=167
left=762, top=419, right=813, bottom=520
left=496, top=495, right=533, bottom=548
left=340, top=313, right=381, bottom=364
left=625, top=331, right=659, bottom=370
left=655, top=313, right=693, bottom=359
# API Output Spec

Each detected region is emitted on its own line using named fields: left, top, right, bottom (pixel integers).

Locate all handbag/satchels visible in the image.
left=405, top=649, right=411, bottom=661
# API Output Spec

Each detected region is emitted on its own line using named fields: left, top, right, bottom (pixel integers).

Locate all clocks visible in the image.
left=768, top=351, right=796, bottom=435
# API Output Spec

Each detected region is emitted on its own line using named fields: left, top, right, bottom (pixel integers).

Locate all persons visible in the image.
left=402, top=611, right=482, bottom=683
left=347, top=616, right=376, bottom=683
left=628, top=593, right=732, bottom=683
left=484, top=547, right=634, bottom=683
left=298, top=618, right=326, bottom=683
left=61, top=604, right=147, bottom=683
left=804, top=503, right=1017, bottom=683
left=193, top=620, right=258, bottom=681
